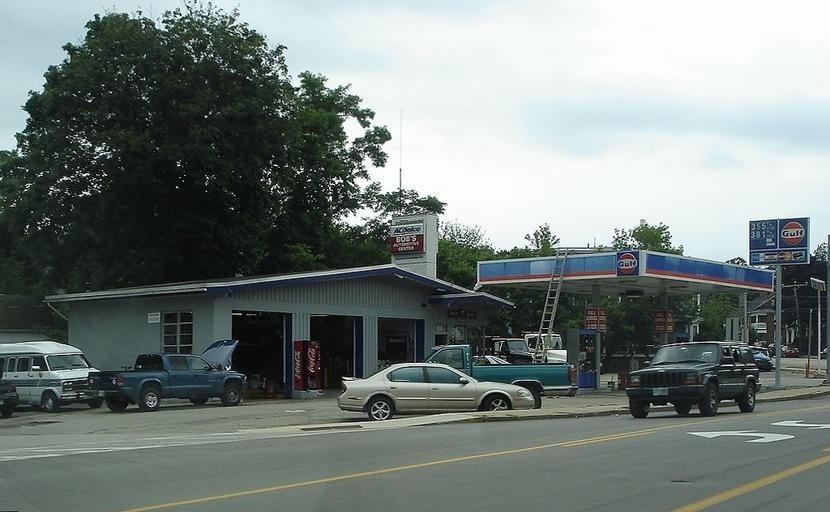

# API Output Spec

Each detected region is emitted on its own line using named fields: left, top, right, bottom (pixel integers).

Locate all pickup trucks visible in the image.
left=84, top=339, right=248, bottom=413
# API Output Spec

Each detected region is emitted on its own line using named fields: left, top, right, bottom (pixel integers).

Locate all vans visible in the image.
left=0, top=340, right=103, bottom=413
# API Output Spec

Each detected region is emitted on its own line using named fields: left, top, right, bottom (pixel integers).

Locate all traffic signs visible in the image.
left=749, top=216, right=811, bottom=264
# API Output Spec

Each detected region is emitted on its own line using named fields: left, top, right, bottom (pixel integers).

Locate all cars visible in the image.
left=455, top=356, right=512, bottom=366
left=781, top=346, right=800, bottom=358
left=337, top=363, right=535, bottom=422
left=1, top=379, right=20, bottom=418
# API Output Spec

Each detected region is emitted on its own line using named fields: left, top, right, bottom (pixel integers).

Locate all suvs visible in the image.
left=748, top=346, right=773, bottom=371
left=524, top=333, right=567, bottom=364
left=624, top=340, right=760, bottom=418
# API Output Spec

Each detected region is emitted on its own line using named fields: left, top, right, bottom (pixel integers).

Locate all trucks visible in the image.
left=474, top=338, right=533, bottom=364
left=387, top=345, right=578, bottom=409
